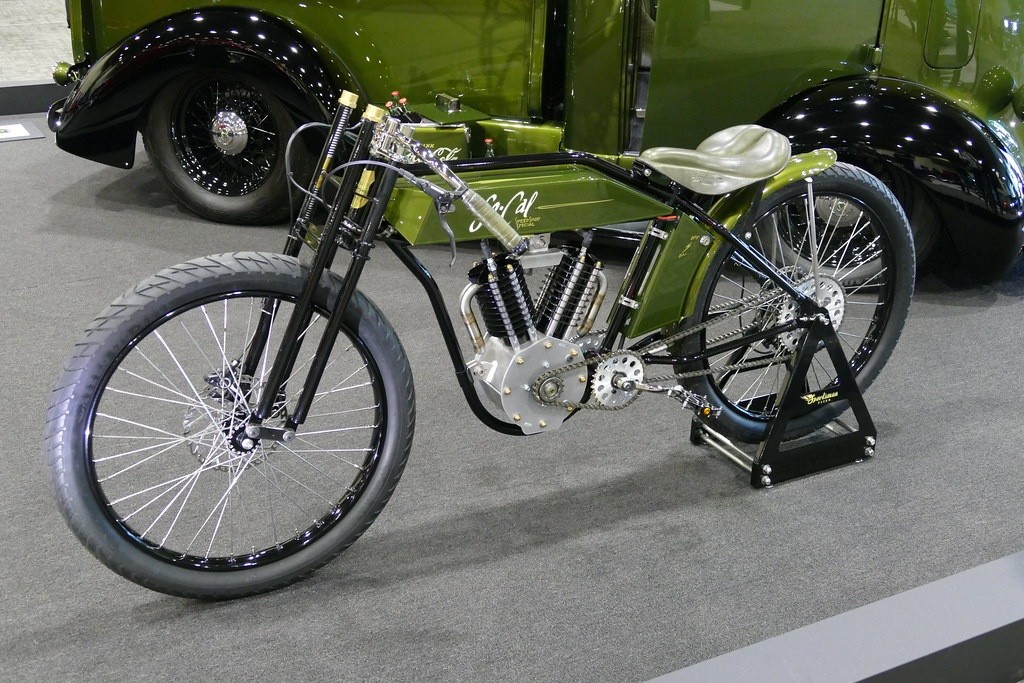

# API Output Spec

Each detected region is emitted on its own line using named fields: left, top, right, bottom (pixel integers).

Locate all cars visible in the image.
left=39, top=0, right=1024, bottom=295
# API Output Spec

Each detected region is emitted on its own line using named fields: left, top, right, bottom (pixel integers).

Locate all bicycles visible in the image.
left=47, top=87, right=922, bottom=604
